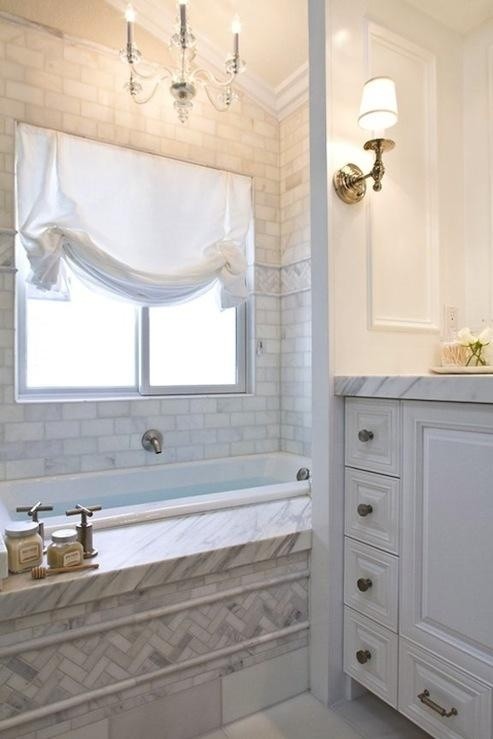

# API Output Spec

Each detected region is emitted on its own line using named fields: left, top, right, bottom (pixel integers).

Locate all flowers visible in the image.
left=454, top=326, right=492, bottom=366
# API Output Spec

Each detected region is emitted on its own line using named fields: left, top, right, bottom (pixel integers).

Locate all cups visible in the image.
left=440, top=339, right=468, bottom=367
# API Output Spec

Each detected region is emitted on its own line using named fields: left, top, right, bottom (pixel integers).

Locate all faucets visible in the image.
left=141, top=429, right=165, bottom=455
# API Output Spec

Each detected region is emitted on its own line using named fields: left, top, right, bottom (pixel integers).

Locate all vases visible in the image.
left=462, top=345, right=486, bottom=366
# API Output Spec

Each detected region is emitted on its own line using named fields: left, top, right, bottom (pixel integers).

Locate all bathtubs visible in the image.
left=0, top=449, right=315, bottom=542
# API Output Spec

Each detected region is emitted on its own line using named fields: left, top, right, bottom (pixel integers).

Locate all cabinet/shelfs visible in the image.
left=329, top=369, right=493, bottom=739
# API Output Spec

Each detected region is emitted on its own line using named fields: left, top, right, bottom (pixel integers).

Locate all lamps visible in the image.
left=107, top=1, right=252, bottom=124
left=329, top=73, right=402, bottom=206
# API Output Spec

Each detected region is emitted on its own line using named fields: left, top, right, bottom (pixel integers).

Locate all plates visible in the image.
left=430, top=366, right=493, bottom=373
